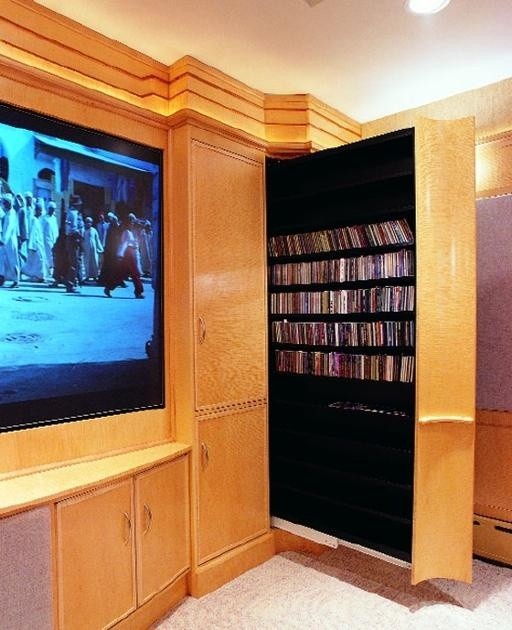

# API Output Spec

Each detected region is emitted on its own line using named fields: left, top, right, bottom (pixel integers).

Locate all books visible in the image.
left=268, top=219, right=414, bottom=383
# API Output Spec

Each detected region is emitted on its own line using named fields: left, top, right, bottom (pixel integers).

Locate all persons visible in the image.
left=1, top=177, right=154, bottom=298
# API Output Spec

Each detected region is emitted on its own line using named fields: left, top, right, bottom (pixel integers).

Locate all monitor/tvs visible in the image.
left=0, top=98, right=164, bottom=434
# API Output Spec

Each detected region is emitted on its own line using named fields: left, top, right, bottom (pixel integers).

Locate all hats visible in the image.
left=70, top=194, right=82, bottom=206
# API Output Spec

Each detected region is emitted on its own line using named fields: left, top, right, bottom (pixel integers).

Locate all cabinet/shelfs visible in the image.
left=34, top=436, right=194, bottom=629
left=258, top=113, right=481, bottom=587
left=163, top=136, right=276, bottom=599
